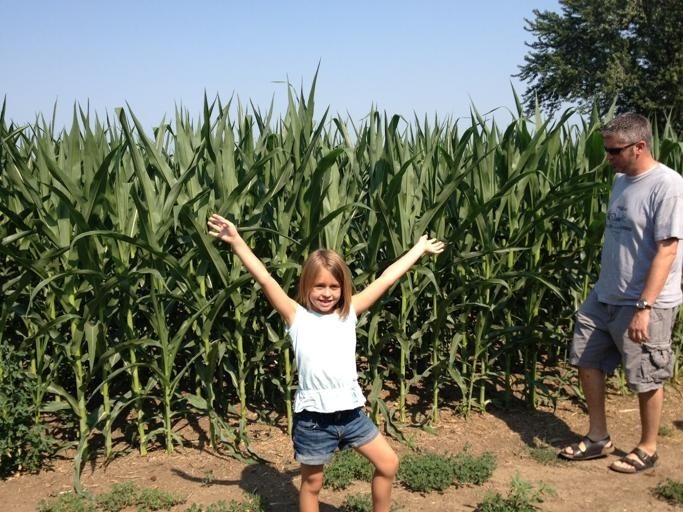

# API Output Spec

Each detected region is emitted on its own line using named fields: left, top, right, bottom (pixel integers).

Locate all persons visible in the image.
left=203, top=213, right=446, bottom=511
left=555, top=111, right=682, bottom=475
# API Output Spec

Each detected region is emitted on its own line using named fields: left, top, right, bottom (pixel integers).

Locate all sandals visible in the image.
left=560, top=434, right=615, bottom=460
left=610, top=447, right=661, bottom=473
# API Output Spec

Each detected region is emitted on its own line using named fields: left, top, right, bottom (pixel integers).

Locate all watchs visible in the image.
left=633, top=298, right=653, bottom=312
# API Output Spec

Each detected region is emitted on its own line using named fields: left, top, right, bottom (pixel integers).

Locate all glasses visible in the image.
left=604, top=142, right=638, bottom=155
